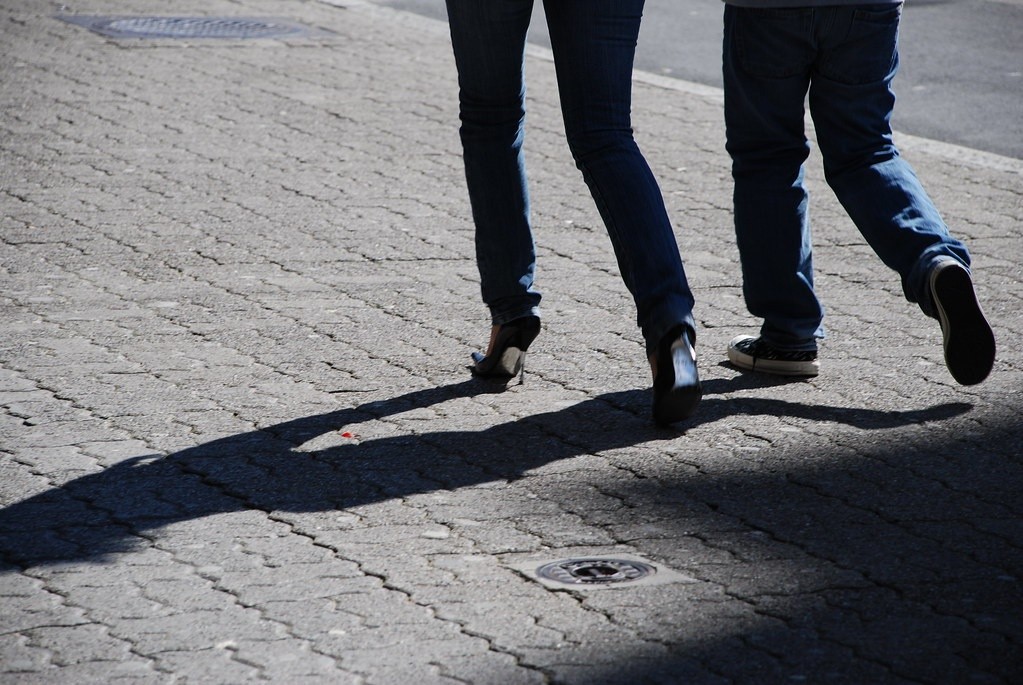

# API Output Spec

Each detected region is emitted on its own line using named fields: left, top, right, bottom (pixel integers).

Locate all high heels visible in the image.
left=653, top=321, right=702, bottom=426
left=471, top=315, right=540, bottom=385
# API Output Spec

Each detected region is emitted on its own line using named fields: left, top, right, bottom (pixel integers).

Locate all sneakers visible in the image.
left=728, top=335, right=819, bottom=376
left=923, top=255, right=996, bottom=386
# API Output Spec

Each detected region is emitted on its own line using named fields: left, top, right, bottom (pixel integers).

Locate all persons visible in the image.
left=722, top=0, right=997, bottom=386
left=443, top=0, right=702, bottom=422
